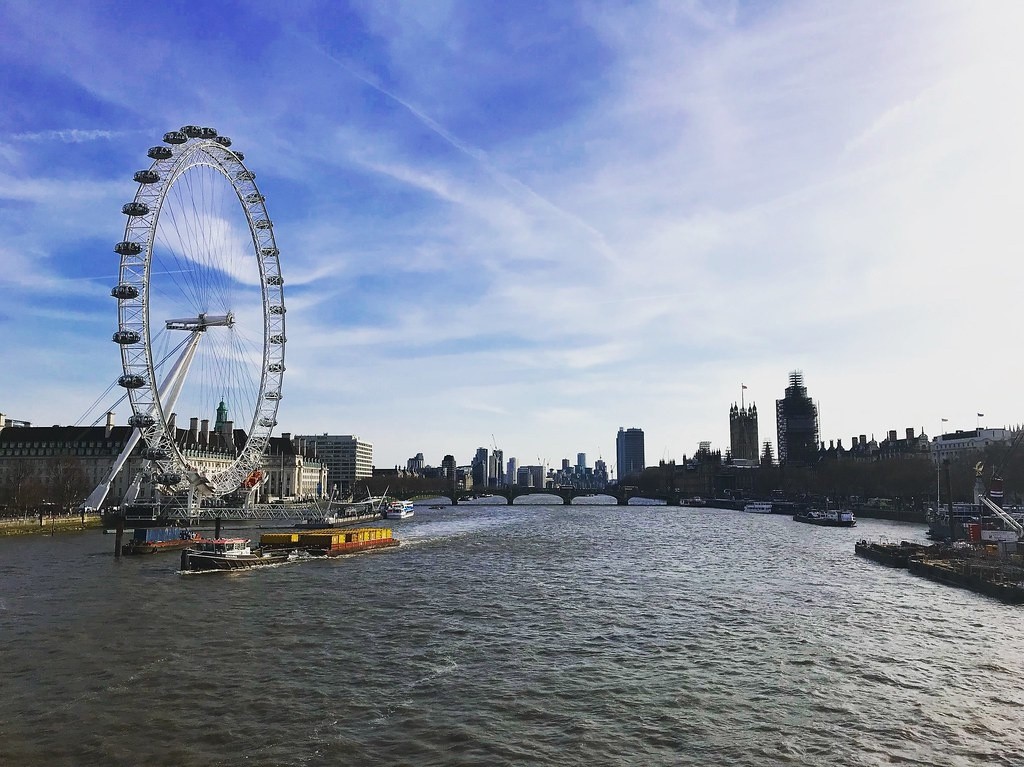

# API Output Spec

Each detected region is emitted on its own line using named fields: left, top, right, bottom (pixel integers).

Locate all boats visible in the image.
left=744, top=502, right=772, bottom=514
left=122, top=526, right=201, bottom=555
left=680, top=495, right=706, bottom=506
left=386, top=500, right=414, bottom=518
left=180, top=547, right=289, bottom=571
left=190, top=537, right=251, bottom=551
left=792, top=510, right=857, bottom=527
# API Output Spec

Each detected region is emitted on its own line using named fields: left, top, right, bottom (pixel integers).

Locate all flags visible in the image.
left=978, top=413, right=984, bottom=417
left=742, top=385, right=747, bottom=389
left=942, top=418, right=948, bottom=422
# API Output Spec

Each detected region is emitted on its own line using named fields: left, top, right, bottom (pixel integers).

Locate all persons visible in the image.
left=180, top=527, right=198, bottom=539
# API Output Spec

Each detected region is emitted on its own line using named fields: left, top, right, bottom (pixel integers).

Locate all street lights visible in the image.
left=977, top=412, right=984, bottom=437
left=941, top=417, right=949, bottom=441
